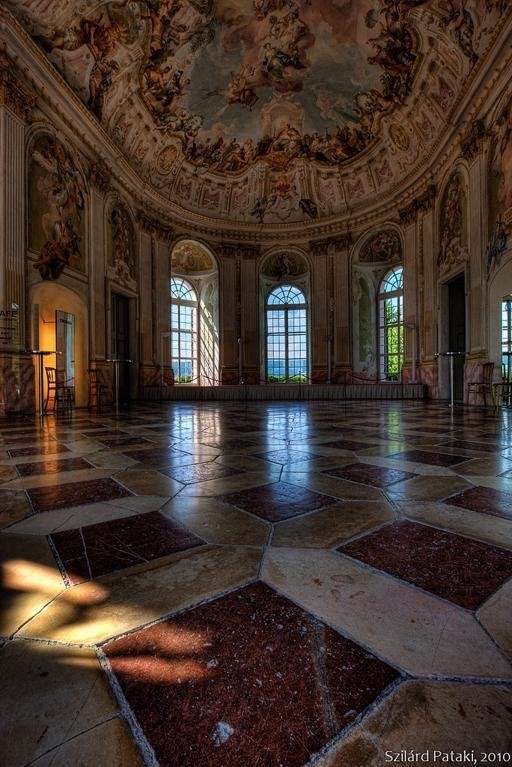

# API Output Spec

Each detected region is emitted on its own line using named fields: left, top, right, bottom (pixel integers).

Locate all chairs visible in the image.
left=467, top=361, right=496, bottom=409
left=86, top=369, right=108, bottom=414
left=492, top=383, right=512, bottom=410
left=45, top=367, right=72, bottom=417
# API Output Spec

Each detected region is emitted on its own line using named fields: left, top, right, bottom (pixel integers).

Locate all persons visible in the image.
left=44, top=0, right=490, bottom=177
left=178, top=244, right=197, bottom=270
left=275, top=251, right=298, bottom=276
left=364, top=230, right=403, bottom=260
left=30, top=144, right=137, bottom=283
left=437, top=98, right=510, bottom=279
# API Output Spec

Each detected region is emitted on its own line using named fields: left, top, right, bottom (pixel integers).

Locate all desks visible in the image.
left=440, top=351, right=465, bottom=416
left=106, top=359, right=131, bottom=417
left=21, top=350, right=56, bottom=416
